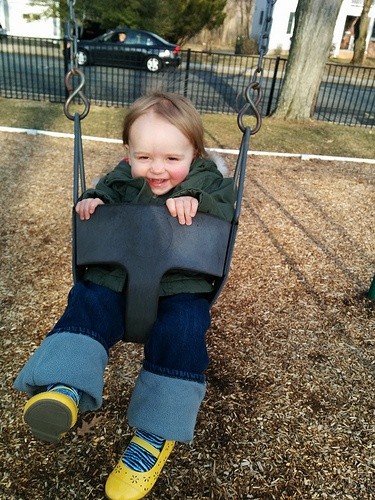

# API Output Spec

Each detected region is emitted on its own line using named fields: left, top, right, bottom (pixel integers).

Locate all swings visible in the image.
left=65, top=0, right=276, bottom=343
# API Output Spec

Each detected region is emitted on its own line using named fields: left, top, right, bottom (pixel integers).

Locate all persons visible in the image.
left=22, top=93, right=239, bottom=500
left=119, top=33, right=126, bottom=42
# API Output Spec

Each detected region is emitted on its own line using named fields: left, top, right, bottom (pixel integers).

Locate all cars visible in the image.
left=64, top=28, right=183, bottom=74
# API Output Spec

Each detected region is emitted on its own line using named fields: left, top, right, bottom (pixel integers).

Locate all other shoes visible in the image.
left=105, top=435, right=176, bottom=500
left=24, top=386, right=78, bottom=443
